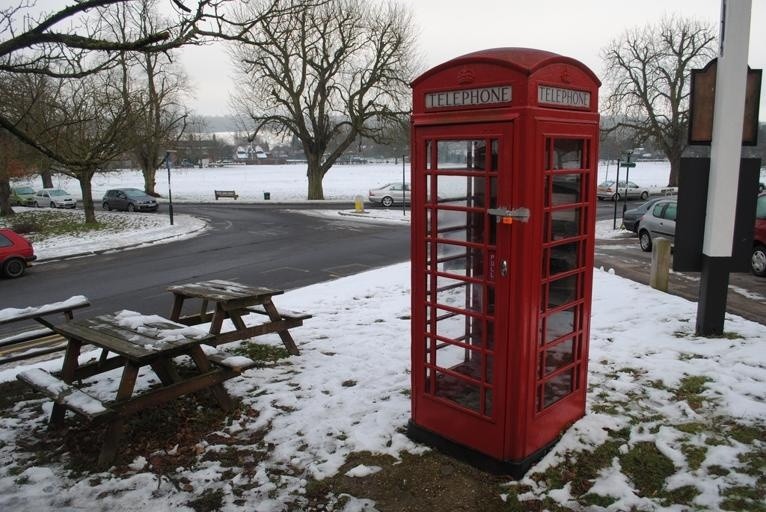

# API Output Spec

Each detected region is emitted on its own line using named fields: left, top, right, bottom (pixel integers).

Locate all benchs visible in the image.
left=195, top=342, right=255, bottom=377
left=14, top=359, right=114, bottom=430
left=213, top=188, right=240, bottom=201
left=245, top=301, right=313, bottom=334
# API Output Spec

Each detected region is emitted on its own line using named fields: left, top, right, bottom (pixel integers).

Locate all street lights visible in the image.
left=163, top=148, right=178, bottom=226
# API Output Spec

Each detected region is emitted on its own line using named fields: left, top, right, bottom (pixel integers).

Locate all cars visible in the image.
left=596, top=177, right=650, bottom=203
left=7, top=184, right=37, bottom=206
left=0, top=227, right=38, bottom=280
left=100, top=187, right=159, bottom=213
left=32, top=186, right=77, bottom=209
left=636, top=198, right=677, bottom=253
left=750, top=191, right=766, bottom=278
left=366, top=180, right=444, bottom=208
left=621, top=195, right=677, bottom=233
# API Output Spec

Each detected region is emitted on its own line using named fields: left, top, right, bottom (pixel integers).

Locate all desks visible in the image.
left=50, top=310, right=235, bottom=438
left=172, top=274, right=301, bottom=361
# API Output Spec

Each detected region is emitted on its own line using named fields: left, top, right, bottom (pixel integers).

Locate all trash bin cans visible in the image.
left=264, top=192, right=270, bottom=201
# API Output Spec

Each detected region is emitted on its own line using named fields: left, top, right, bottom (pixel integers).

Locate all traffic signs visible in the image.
left=620, top=163, right=636, bottom=168
